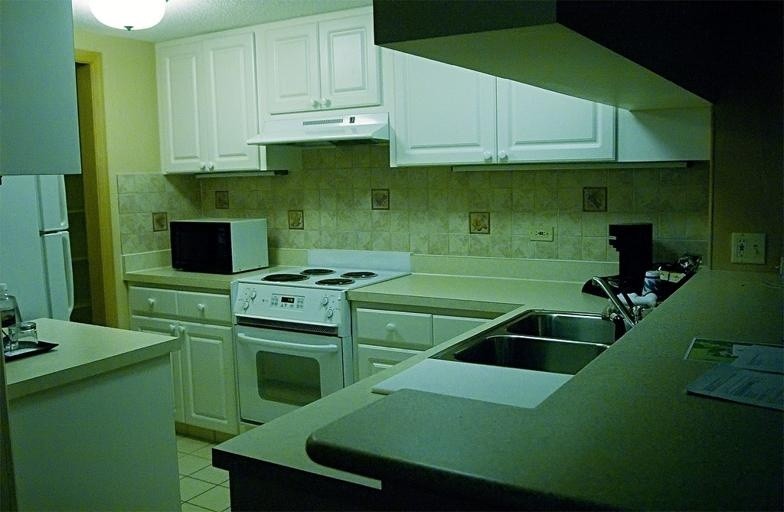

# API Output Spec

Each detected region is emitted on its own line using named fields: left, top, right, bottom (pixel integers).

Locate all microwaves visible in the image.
left=167, top=218, right=269, bottom=275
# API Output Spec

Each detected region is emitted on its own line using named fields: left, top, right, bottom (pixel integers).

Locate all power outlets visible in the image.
left=731, top=232, right=766, bottom=265
left=531, top=224, right=556, bottom=241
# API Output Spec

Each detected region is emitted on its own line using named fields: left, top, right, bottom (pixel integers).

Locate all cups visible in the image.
left=18, top=322, right=38, bottom=348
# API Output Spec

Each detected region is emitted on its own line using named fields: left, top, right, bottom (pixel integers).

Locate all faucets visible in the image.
left=607, top=277, right=642, bottom=320
left=592, top=276, right=634, bottom=329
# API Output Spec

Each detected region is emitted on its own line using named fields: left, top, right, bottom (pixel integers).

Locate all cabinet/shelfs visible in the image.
left=266, top=6, right=382, bottom=115
left=433, top=298, right=494, bottom=351
left=128, top=272, right=239, bottom=444
left=155, top=28, right=295, bottom=178
left=357, top=290, right=432, bottom=382
left=1, top=317, right=183, bottom=512
left=616, top=110, right=714, bottom=162
left=211, top=306, right=655, bottom=512
left=0, top=0, right=82, bottom=175
left=392, top=50, right=617, bottom=167
left=375, top=1, right=709, bottom=112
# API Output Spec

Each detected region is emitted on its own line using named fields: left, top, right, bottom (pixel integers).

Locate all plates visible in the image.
left=0, top=333, right=59, bottom=359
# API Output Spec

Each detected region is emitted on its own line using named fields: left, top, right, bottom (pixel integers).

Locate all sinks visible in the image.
left=496, top=308, right=629, bottom=346
left=426, top=333, right=609, bottom=375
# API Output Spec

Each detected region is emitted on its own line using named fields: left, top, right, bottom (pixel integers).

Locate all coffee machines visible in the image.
left=581, top=218, right=655, bottom=296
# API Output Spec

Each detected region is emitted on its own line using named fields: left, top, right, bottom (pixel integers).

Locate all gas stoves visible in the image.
left=230, top=265, right=413, bottom=338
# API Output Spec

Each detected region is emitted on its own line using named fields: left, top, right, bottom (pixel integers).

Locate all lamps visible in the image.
left=87, top=0, right=167, bottom=31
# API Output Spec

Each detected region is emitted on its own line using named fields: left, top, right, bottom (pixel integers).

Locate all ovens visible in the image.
left=234, top=326, right=346, bottom=425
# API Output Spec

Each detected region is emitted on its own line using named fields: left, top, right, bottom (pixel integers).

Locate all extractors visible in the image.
left=246, top=113, right=390, bottom=146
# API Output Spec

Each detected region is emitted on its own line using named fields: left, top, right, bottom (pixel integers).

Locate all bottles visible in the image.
left=0, top=283, right=23, bottom=354
